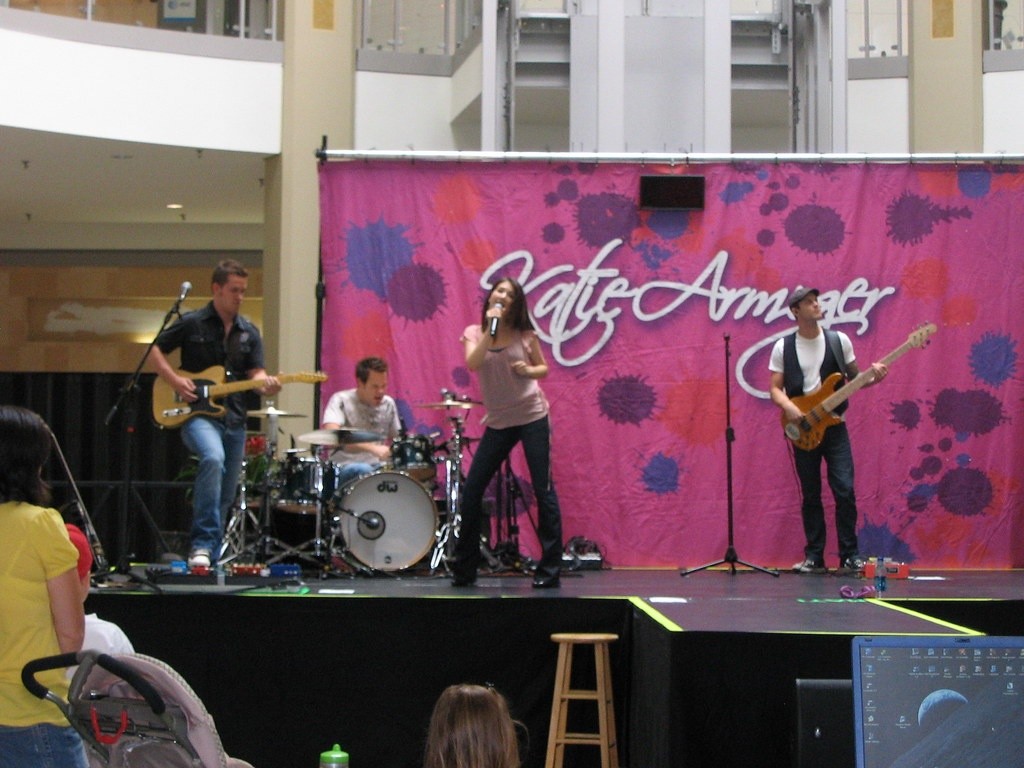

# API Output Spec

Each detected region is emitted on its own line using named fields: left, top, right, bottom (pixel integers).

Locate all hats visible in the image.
left=789, top=288, right=819, bottom=308
left=66, top=523, right=94, bottom=580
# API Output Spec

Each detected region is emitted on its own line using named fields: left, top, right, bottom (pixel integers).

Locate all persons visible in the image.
left=451, top=277, right=564, bottom=588
left=322, top=355, right=403, bottom=500
left=150, top=259, right=283, bottom=567
left=424, top=683, right=520, bottom=768
left=768, top=288, right=889, bottom=573
left=0, top=404, right=145, bottom=768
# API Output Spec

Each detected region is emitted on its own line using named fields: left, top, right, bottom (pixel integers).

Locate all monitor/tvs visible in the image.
left=852, top=635, right=1024, bottom=768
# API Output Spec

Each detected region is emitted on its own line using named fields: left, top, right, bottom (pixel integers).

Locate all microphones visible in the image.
left=399, top=417, right=408, bottom=432
left=489, top=302, right=503, bottom=336
left=367, top=518, right=380, bottom=529
left=179, top=281, right=192, bottom=300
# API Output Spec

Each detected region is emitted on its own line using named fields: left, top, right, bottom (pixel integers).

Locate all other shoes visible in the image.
left=188, top=549, right=212, bottom=567
left=452, top=573, right=477, bottom=586
left=793, top=560, right=826, bottom=573
left=533, top=576, right=559, bottom=588
left=841, top=558, right=865, bottom=570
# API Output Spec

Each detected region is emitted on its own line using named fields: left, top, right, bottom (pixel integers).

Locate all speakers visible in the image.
left=639, top=174, right=705, bottom=211
left=779, top=678, right=857, bottom=768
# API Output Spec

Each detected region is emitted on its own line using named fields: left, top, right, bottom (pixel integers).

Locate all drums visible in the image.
left=324, top=472, right=438, bottom=575
left=273, top=459, right=336, bottom=513
left=389, top=434, right=439, bottom=480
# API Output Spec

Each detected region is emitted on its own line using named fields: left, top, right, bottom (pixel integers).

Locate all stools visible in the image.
left=544, top=633, right=622, bottom=768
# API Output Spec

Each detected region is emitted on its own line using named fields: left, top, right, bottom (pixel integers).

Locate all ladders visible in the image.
left=545, top=633, right=619, bottom=767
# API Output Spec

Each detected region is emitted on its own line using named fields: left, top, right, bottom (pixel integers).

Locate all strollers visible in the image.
left=21, top=649, right=255, bottom=768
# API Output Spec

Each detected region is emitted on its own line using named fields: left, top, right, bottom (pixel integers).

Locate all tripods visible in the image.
left=83, top=286, right=187, bottom=592
left=427, top=409, right=538, bottom=578
left=216, top=400, right=373, bottom=581
left=680, top=333, right=779, bottom=577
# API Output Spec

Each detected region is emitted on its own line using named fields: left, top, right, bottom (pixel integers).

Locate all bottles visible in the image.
left=874, top=556, right=887, bottom=595
left=319, top=744, right=349, bottom=768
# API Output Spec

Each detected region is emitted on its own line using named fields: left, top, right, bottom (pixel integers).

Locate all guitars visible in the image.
left=152, top=363, right=328, bottom=428
left=779, top=319, right=938, bottom=452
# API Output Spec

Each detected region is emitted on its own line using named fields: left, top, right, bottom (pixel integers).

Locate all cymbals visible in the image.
left=248, top=407, right=308, bottom=418
left=420, top=399, right=484, bottom=409
left=296, top=428, right=381, bottom=446
